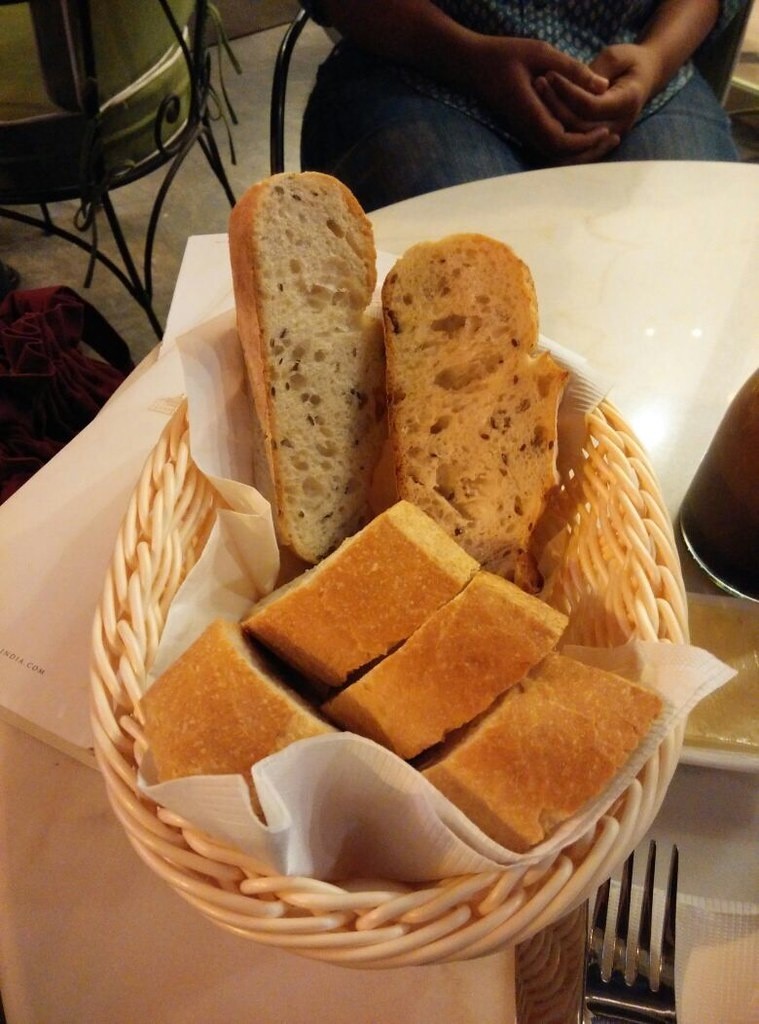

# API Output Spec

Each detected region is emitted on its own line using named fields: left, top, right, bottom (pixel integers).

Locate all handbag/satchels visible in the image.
left=0, top=284, right=135, bottom=507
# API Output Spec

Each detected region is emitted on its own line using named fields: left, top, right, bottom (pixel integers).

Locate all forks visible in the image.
left=581, top=841, right=682, bottom=1020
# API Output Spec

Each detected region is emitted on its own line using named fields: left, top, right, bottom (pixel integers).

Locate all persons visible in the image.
left=300, top=0, right=740, bottom=213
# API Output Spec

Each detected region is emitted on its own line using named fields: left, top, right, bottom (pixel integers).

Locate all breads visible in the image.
left=138, top=498, right=668, bottom=848
left=378, top=234, right=569, bottom=594
left=228, top=172, right=378, bottom=567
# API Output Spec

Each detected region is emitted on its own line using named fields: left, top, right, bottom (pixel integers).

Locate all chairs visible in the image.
left=0, top=0, right=759, bottom=345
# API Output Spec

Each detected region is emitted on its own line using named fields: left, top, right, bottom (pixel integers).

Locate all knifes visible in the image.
left=516, top=901, right=592, bottom=1022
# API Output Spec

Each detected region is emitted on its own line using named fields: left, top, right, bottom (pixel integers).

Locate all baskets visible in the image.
left=91, top=333, right=687, bottom=968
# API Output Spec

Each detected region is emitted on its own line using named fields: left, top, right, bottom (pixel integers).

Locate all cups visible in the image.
left=680, top=366, right=759, bottom=600
